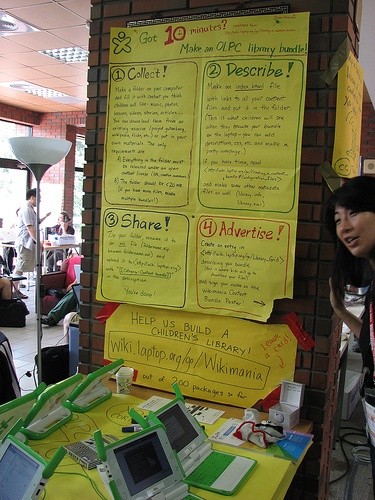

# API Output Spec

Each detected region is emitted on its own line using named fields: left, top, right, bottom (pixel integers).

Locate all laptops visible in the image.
left=0, top=358, right=257, bottom=500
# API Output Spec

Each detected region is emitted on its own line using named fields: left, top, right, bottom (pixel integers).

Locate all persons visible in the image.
left=325, top=176, right=375, bottom=476
left=11, top=188, right=50, bottom=298
left=40, top=280, right=78, bottom=326
left=0, top=278, right=11, bottom=299
left=47, top=212, right=75, bottom=273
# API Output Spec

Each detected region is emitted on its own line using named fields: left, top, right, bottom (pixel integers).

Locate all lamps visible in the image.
left=7, top=136, right=72, bottom=385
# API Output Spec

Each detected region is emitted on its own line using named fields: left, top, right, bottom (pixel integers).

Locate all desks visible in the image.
left=0, top=239, right=81, bottom=291
left=0, top=374, right=314, bottom=500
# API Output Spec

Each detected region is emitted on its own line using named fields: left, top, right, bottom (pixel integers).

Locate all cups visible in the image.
left=116, top=367, right=134, bottom=394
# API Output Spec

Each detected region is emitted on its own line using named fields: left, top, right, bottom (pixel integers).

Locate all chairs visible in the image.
left=0, top=255, right=27, bottom=302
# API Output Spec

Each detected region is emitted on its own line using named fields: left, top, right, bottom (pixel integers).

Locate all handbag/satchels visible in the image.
left=41, top=295, right=60, bottom=316
left=0, top=298, right=30, bottom=328
left=41, top=317, right=57, bottom=327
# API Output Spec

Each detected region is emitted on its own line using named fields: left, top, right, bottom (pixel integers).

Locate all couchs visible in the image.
left=35, top=256, right=82, bottom=316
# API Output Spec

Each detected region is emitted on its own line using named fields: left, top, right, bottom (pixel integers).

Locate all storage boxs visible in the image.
left=337, top=369, right=365, bottom=421
left=269, top=380, right=305, bottom=430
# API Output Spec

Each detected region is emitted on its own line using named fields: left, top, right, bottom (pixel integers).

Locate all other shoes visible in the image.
left=13, top=290, right=28, bottom=299
